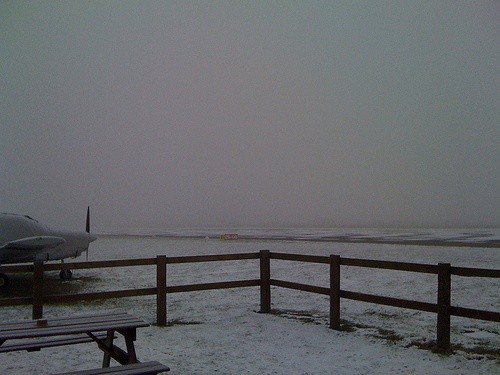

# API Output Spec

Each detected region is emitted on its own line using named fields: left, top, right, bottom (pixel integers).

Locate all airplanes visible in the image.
left=0, top=205, right=97, bottom=288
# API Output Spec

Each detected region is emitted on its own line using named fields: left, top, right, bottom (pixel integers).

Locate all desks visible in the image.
left=1, top=311, right=145, bottom=368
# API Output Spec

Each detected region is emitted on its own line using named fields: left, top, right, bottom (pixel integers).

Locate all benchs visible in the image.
left=0, top=333, right=116, bottom=351
left=56, top=361, right=170, bottom=375
